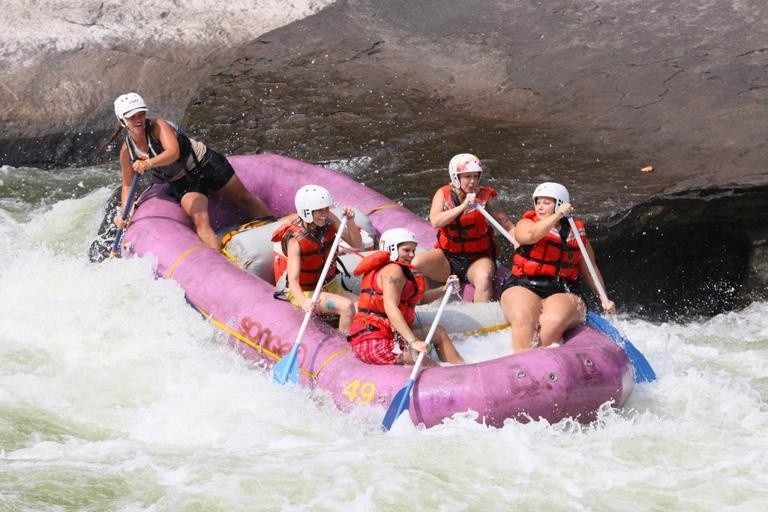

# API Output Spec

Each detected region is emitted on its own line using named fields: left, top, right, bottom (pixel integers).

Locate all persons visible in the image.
left=114, top=92, right=276, bottom=252
left=498, top=181, right=616, bottom=354
left=269, top=184, right=362, bottom=336
left=409, top=151, right=521, bottom=304
left=347, top=228, right=466, bottom=368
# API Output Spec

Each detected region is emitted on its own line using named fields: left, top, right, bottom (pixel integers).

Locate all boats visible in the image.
left=115, top=149, right=638, bottom=432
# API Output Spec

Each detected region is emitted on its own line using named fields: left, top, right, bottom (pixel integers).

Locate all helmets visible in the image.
left=294, top=185, right=334, bottom=225
left=113, top=92, right=147, bottom=128
left=379, top=228, right=419, bottom=262
left=533, top=182, right=570, bottom=216
left=450, top=152, right=484, bottom=190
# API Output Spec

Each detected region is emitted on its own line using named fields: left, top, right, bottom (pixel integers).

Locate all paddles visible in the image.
left=381, top=280, right=454, bottom=433
left=271, top=215, right=348, bottom=384
left=469, top=201, right=656, bottom=383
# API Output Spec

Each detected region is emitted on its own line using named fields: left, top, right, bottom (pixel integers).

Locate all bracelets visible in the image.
left=145, top=160, right=153, bottom=169
left=438, top=285, right=445, bottom=293
left=407, top=336, right=418, bottom=348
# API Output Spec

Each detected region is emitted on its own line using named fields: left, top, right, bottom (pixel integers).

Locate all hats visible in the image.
left=124, top=107, right=148, bottom=119
